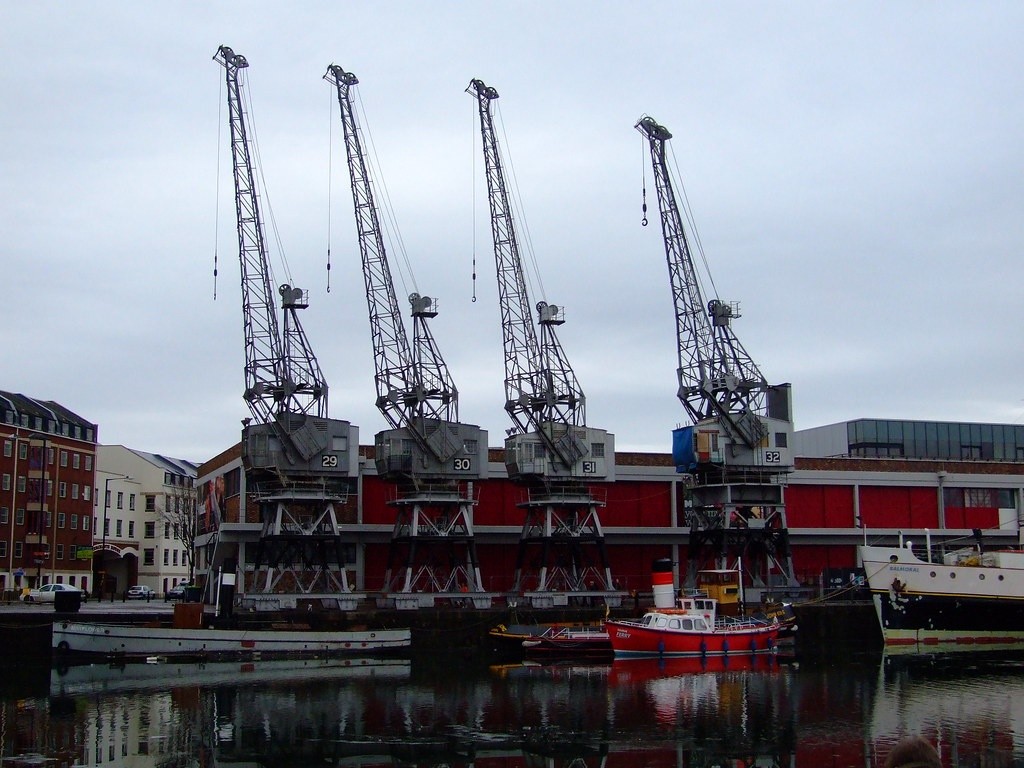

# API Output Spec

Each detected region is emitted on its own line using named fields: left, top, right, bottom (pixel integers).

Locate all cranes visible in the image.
left=213, top=47, right=360, bottom=592
left=466, top=77, right=614, bottom=596
left=324, top=63, right=488, bottom=593
left=638, top=118, right=803, bottom=589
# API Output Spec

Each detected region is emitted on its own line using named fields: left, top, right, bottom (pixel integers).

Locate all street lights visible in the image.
left=97, top=476, right=142, bottom=604
left=30, top=432, right=60, bottom=584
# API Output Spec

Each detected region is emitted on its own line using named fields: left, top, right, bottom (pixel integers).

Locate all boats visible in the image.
left=606, top=655, right=779, bottom=739
left=520, top=624, right=797, bottom=652
left=605, top=597, right=780, bottom=660
left=48, top=656, right=411, bottom=699
left=490, top=621, right=609, bottom=639
left=693, top=570, right=799, bottom=639
left=50, top=617, right=411, bottom=654
left=858, top=527, right=1024, bottom=665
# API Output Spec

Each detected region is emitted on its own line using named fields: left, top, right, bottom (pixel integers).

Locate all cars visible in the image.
left=19, top=583, right=86, bottom=604
left=128, top=586, right=156, bottom=600
left=167, top=586, right=185, bottom=600
